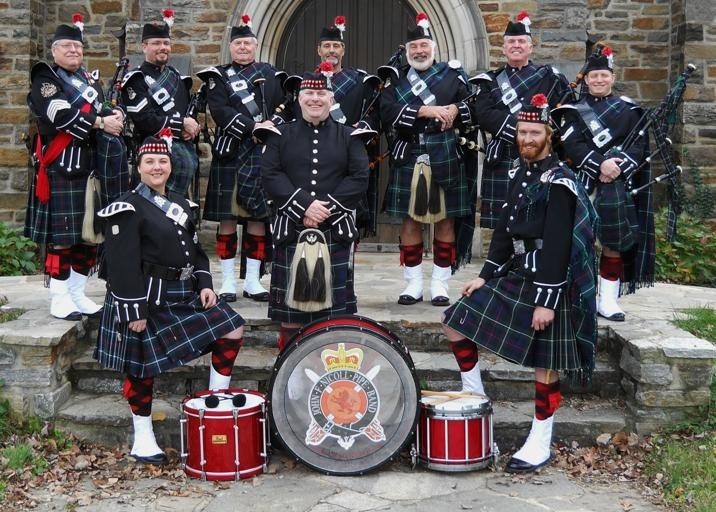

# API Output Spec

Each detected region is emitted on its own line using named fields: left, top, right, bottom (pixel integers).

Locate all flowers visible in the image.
left=334, top=15, right=346, bottom=39
left=72, top=13, right=84, bottom=33
left=603, top=46, right=613, bottom=68
left=517, top=11, right=531, bottom=33
left=320, top=61, right=335, bottom=88
left=162, top=8, right=174, bottom=28
left=415, top=12, right=430, bottom=37
left=240, top=14, right=252, bottom=28
left=158, top=127, right=173, bottom=154
left=530, top=93, right=549, bottom=121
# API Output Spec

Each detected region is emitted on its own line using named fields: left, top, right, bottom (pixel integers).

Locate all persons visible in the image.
left=92, top=134, right=246, bottom=464
left=195, top=14, right=287, bottom=302
left=250, top=61, right=369, bottom=348
left=375, top=10, right=479, bottom=306
left=440, top=93, right=599, bottom=473
left=115, top=10, right=202, bottom=228
left=281, top=15, right=383, bottom=238
left=19, top=14, right=126, bottom=321
left=468, top=12, right=574, bottom=231
left=550, top=42, right=656, bottom=320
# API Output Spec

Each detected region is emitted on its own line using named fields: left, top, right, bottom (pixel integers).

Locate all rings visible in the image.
left=198, top=124, right=201, bottom=127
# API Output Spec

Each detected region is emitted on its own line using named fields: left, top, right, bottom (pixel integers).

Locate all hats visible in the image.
left=585, top=52, right=615, bottom=76
left=134, top=137, right=170, bottom=156
left=318, top=26, right=345, bottom=44
left=299, top=71, right=335, bottom=91
left=51, top=22, right=84, bottom=44
left=229, top=25, right=258, bottom=44
left=502, top=21, right=533, bottom=40
left=517, top=102, right=553, bottom=126
left=405, top=26, right=434, bottom=44
left=142, top=22, right=170, bottom=41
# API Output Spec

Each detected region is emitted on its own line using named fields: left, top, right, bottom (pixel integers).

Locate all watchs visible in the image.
left=98, top=116, right=105, bottom=132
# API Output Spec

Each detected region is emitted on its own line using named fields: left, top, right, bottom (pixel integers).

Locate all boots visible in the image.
left=243, top=258, right=271, bottom=301
left=431, top=263, right=452, bottom=306
left=217, top=257, right=237, bottom=302
left=50, top=264, right=106, bottom=321
left=596, top=276, right=627, bottom=321
left=435, top=357, right=491, bottom=414
left=505, top=412, right=556, bottom=475
left=129, top=409, right=169, bottom=466
left=399, top=262, right=425, bottom=303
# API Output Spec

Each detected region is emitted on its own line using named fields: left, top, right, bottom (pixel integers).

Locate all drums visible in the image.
left=410, top=392, right=499, bottom=473
left=179, top=388, right=270, bottom=483
left=270, top=315, right=420, bottom=474
